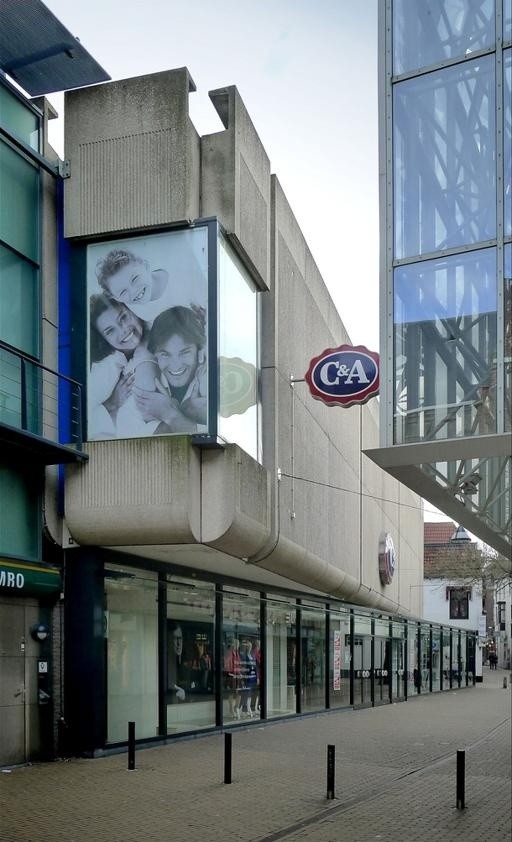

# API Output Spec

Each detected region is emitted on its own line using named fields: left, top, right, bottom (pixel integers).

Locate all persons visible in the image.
left=166, top=621, right=191, bottom=702
left=87, top=247, right=208, bottom=441
left=488, top=653, right=494, bottom=670
left=493, top=653, right=498, bottom=670
left=222, top=635, right=261, bottom=721
left=199, top=649, right=212, bottom=690
